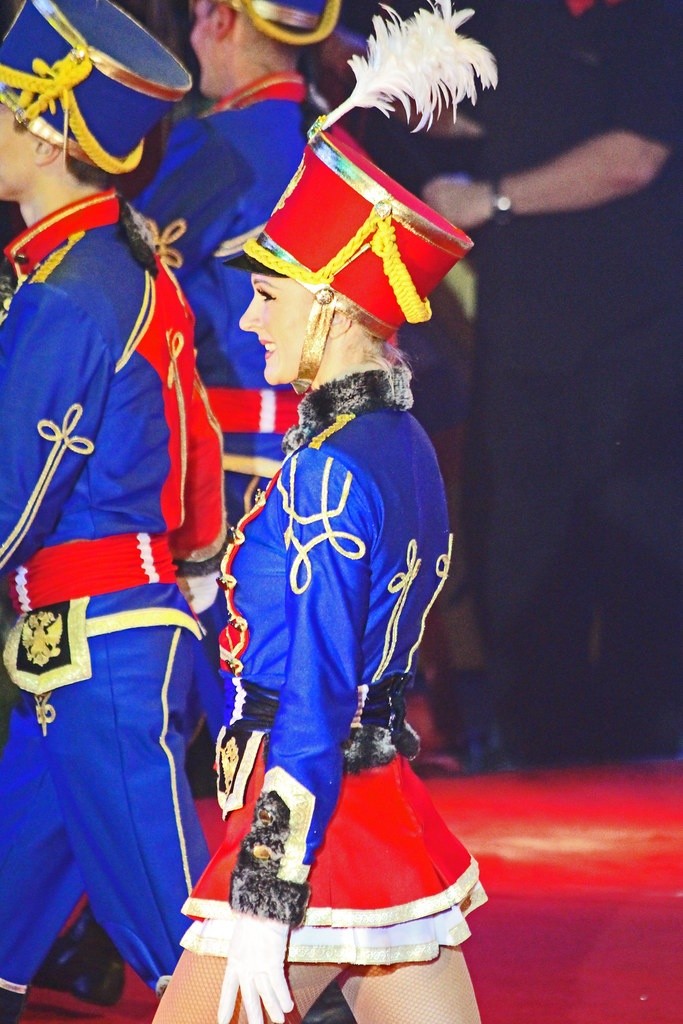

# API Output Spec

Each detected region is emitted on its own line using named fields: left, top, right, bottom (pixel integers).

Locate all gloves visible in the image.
left=185, top=570, right=220, bottom=615
left=217, top=913, right=296, bottom=1024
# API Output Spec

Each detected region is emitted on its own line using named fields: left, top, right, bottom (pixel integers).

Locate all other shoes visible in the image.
left=32, top=898, right=123, bottom=1007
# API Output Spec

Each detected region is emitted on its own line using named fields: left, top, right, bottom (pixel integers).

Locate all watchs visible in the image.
left=489, top=175, right=512, bottom=227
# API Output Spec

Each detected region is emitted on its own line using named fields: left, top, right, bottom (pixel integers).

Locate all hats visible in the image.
left=220, top=0, right=341, bottom=45
left=1, top=0, right=193, bottom=176
left=211, top=0, right=498, bottom=340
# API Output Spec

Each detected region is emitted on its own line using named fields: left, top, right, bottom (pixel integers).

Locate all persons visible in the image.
left=320, top=1, right=683, bottom=761
left=151, top=132, right=491, bottom=1024
left=127, top=1, right=345, bottom=736
left=0, top=1, right=226, bottom=1024
